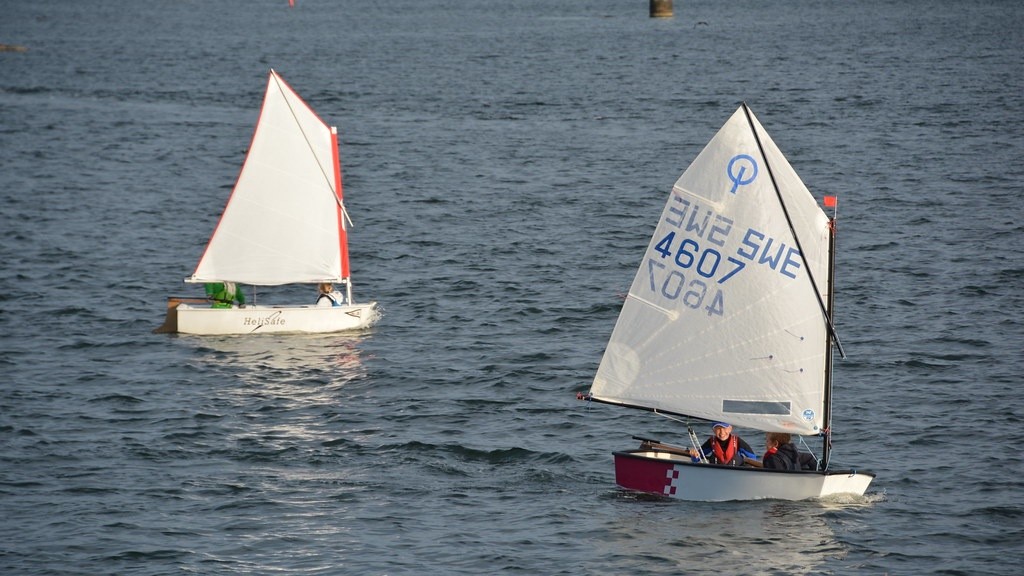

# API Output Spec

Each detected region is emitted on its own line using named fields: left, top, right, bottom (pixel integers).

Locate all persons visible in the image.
left=743, top=432, right=818, bottom=471
left=315, top=283, right=344, bottom=307
left=688, top=422, right=753, bottom=465
left=203, top=283, right=246, bottom=308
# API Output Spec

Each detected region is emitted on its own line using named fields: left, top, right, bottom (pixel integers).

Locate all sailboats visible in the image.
left=153, top=69, right=379, bottom=336
left=578, top=101, right=876, bottom=504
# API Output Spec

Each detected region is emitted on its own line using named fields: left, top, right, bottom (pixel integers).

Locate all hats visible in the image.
left=712, top=420, right=729, bottom=430
